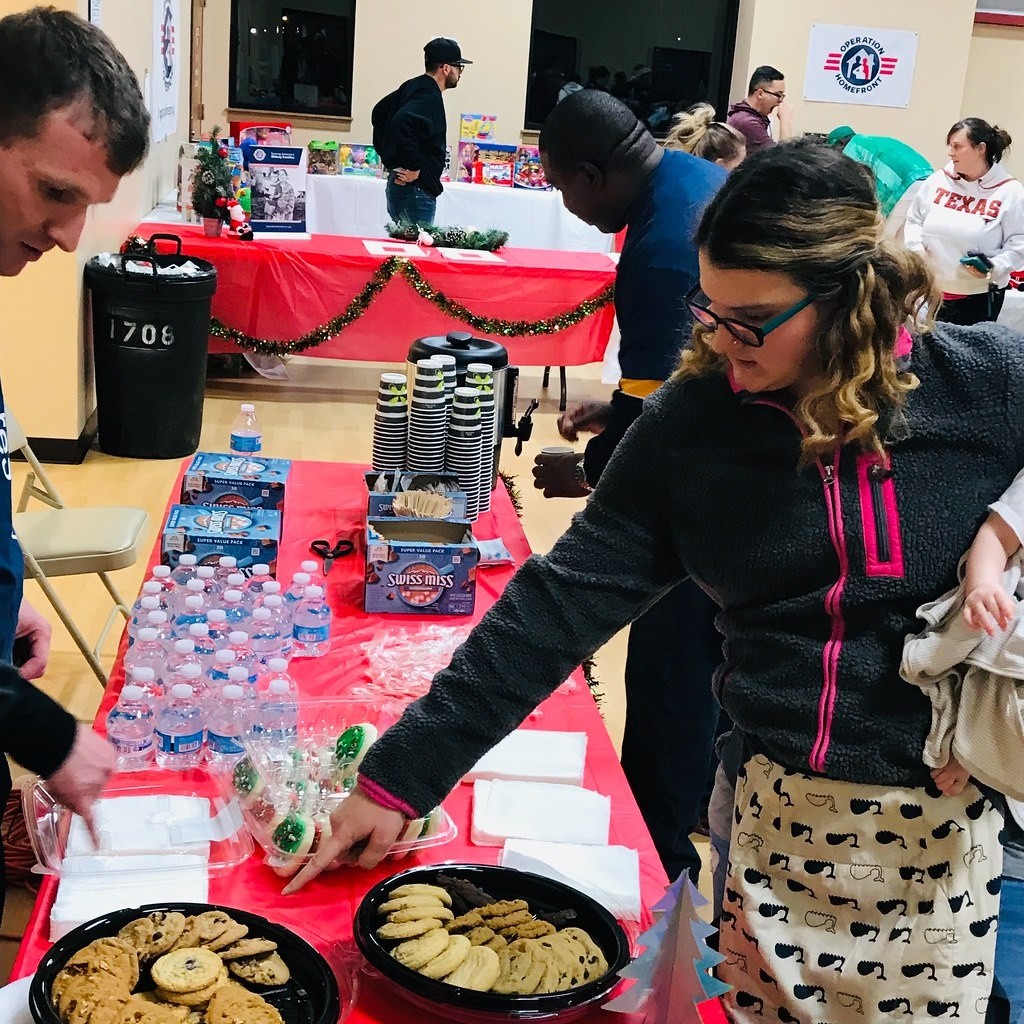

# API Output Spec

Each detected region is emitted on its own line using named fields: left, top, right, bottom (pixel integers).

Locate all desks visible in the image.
left=304, top=174, right=617, bottom=255
left=9, top=457, right=729, bottom=1024
left=120, top=222, right=619, bottom=411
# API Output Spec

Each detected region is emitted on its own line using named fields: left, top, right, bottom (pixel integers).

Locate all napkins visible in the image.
left=460, top=729, right=640, bottom=922
left=0, top=972, right=36, bottom=1024
left=47, top=796, right=212, bottom=944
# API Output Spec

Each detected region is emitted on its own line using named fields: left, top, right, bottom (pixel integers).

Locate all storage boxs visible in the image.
left=179, top=452, right=291, bottom=544
left=456, top=113, right=554, bottom=192
left=219, top=121, right=293, bottom=147
left=363, top=471, right=478, bottom=615
left=161, top=504, right=283, bottom=580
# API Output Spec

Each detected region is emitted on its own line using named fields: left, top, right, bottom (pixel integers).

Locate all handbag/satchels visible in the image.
left=713, top=752, right=1003, bottom=1024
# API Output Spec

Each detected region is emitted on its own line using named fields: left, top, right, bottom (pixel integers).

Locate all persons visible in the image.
left=263, top=169, right=295, bottom=220
left=663, top=103, right=752, bottom=172
left=280, top=145, right=1023, bottom=1021
left=905, top=118, right=1023, bottom=320
left=1, top=4, right=151, bottom=848
left=521, top=88, right=733, bottom=500
left=826, top=120, right=935, bottom=217
left=733, top=65, right=792, bottom=153
left=370, top=38, right=465, bottom=227
left=588, top=63, right=643, bottom=100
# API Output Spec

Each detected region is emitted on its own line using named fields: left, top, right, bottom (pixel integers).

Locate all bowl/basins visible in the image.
left=29, top=903, right=339, bottom=1024
left=354, top=863, right=633, bottom=1023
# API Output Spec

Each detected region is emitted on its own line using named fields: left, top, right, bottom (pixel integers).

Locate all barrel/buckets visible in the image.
left=404, top=330, right=540, bottom=488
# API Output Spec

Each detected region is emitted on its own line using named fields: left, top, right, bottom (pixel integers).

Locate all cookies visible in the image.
left=375, top=884, right=609, bottom=995
left=52, top=910, right=290, bottom=1024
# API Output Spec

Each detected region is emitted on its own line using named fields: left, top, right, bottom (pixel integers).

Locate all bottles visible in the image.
left=105, top=552, right=332, bottom=777
left=230, top=404, right=261, bottom=457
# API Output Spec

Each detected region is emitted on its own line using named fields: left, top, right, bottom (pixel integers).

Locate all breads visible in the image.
left=233, top=723, right=441, bottom=876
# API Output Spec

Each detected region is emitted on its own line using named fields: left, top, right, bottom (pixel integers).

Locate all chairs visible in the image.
left=2, top=404, right=150, bottom=691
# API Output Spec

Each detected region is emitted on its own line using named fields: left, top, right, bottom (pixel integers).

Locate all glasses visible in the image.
left=754, top=87, right=786, bottom=103
left=682, top=284, right=830, bottom=350
left=440, top=63, right=465, bottom=74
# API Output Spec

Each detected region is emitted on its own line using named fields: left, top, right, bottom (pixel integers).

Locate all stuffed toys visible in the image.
left=227, top=199, right=245, bottom=230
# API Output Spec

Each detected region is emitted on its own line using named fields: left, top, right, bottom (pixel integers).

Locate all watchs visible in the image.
left=574, top=458, right=596, bottom=491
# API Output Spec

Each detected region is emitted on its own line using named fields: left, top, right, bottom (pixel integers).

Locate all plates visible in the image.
left=514, top=177, right=551, bottom=189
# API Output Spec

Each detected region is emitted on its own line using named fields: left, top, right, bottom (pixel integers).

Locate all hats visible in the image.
left=423, top=37, right=474, bottom=64
left=828, top=126, right=856, bottom=148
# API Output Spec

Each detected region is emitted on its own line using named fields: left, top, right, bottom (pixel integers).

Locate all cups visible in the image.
left=541, top=447, right=575, bottom=467
left=393, top=508, right=453, bottom=519
left=371, top=355, right=495, bottom=524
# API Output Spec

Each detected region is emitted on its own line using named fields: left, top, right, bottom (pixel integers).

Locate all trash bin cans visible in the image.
left=86, top=233, right=218, bottom=459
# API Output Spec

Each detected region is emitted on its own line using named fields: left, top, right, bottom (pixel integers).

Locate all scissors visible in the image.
left=311, top=539, right=354, bottom=576
